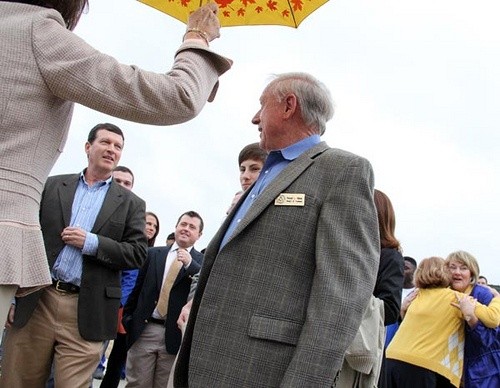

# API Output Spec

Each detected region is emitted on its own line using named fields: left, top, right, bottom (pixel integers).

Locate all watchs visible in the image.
left=183, top=28, right=210, bottom=47
left=465, top=312, right=476, bottom=321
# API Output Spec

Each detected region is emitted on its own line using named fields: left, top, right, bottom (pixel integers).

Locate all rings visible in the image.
left=66, top=235, right=69, bottom=240
left=408, top=299, right=410, bottom=302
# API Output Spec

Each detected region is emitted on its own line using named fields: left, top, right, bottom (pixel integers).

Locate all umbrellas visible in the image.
left=136, top=0, right=328, bottom=30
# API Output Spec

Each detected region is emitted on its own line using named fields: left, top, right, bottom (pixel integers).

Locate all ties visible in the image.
left=155, top=247, right=186, bottom=320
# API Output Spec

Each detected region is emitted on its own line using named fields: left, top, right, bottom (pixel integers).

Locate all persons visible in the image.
left=0, top=0, right=234, bottom=351
left=172, top=74, right=380, bottom=388
left=1, top=124, right=500, bottom=388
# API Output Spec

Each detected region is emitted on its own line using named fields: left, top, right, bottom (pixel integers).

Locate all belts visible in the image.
left=50, top=277, right=81, bottom=294
left=149, top=317, right=165, bottom=325
